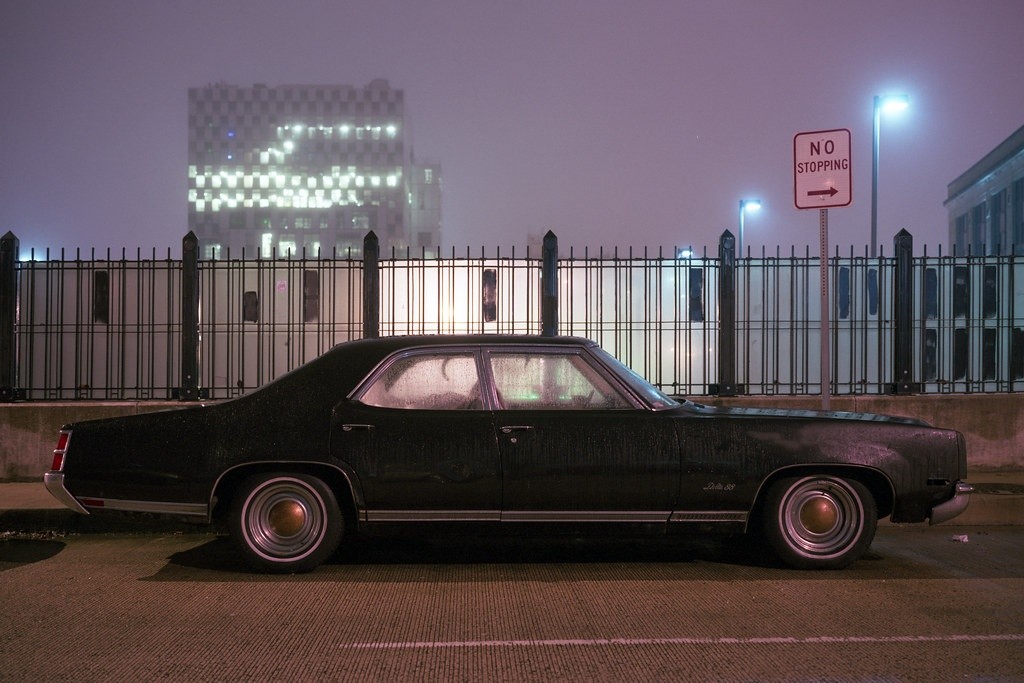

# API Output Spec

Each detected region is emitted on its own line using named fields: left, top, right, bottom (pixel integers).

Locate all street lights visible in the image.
left=739, top=199, right=765, bottom=260
left=869, top=92, right=909, bottom=257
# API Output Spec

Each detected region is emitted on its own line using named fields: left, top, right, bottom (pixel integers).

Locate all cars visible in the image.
left=46, top=331, right=980, bottom=572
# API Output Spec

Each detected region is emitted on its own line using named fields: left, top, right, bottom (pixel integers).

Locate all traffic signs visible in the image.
left=791, top=130, right=854, bottom=211
